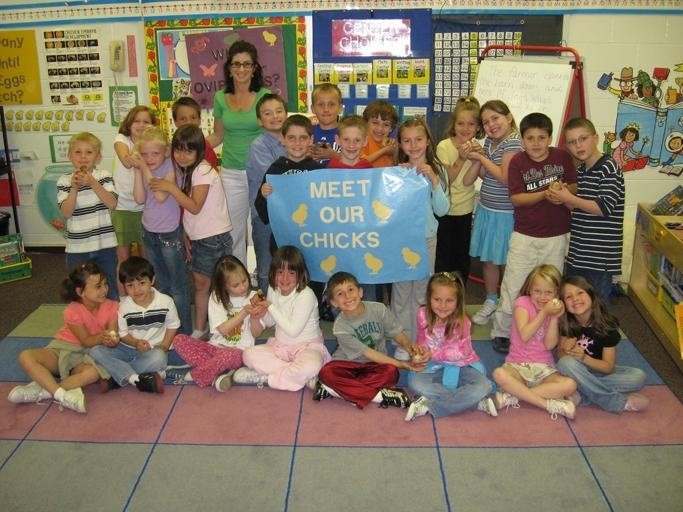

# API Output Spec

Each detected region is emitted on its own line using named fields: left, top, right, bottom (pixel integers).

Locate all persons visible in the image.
left=613, top=123, right=641, bottom=169
left=246, top=94, right=290, bottom=296
left=173, top=255, right=267, bottom=393
left=662, top=131, right=683, bottom=167
left=464, top=100, right=525, bottom=325
left=391, top=118, right=451, bottom=361
left=361, top=100, right=397, bottom=305
left=321, top=114, right=371, bottom=321
left=313, top=271, right=428, bottom=410
left=311, top=84, right=348, bottom=171
left=495, top=265, right=577, bottom=419
left=255, top=115, right=324, bottom=319
left=403, top=272, right=500, bottom=423
left=206, top=41, right=272, bottom=272
left=432, top=96, right=484, bottom=288
left=8, top=261, right=120, bottom=413
left=56, top=134, right=119, bottom=304
left=607, top=67, right=638, bottom=100
left=109, top=105, right=156, bottom=300
left=90, top=256, right=181, bottom=393
left=555, top=268, right=649, bottom=414
left=234, top=245, right=332, bottom=391
left=491, top=114, right=577, bottom=353
left=549, top=118, right=625, bottom=308
left=174, top=97, right=219, bottom=175
left=635, top=70, right=659, bottom=108
left=172, top=125, right=234, bottom=339
left=125, top=126, right=192, bottom=350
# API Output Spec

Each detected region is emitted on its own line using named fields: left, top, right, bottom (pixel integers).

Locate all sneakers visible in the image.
left=491, top=336, right=509, bottom=353
left=311, top=382, right=330, bottom=399
left=477, top=397, right=497, bottom=417
left=492, top=389, right=521, bottom=412
left=7, top=381, right=52, bottom=409
left=565, top=390, right=582, bottom=405
left=232, top=366, right=268, bottom=389
left=404, top=395, right=430, bottom=421
left=135, top=370, right=164, bottom=393
left=212, top=370, right=237, bottom=392
left=548, top=398, right=576, bottom=418
left=379, top=387, right=408, bottom=408
left=471, top=298, right=497, bottom=327
left=52, top=386, right=88, bottom=413
left=98, top=375, right=118, bottom=394
left=157, top=362, right=197, bottom=385
left=624, top=393, right=650, bottom=412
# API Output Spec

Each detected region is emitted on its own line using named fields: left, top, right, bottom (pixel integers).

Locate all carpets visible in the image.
left=1, top=303, right=683, bottom=511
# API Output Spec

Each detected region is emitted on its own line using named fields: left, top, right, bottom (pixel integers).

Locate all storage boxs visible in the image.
left=0, top=233, right=25, bottom=266
left=0, top=257, right=33, bottom=285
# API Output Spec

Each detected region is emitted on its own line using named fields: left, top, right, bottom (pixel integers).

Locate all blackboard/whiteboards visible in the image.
left=470, top=44, right=586, bottom=149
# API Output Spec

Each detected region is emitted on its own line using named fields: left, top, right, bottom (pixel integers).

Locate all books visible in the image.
left=0, top=232, right=25, bottom=267
left=649, top=251, right=683, bottom=322
left=651, top=183, right=683, bottom=230
left=0, top=172, right=20, bottom=210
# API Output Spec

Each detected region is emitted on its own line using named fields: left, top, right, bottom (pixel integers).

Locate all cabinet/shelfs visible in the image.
left=628, top=201, right=683, bottom=376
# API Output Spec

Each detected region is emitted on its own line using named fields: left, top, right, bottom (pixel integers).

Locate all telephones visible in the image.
left=108, top=40, right=125, bottom=76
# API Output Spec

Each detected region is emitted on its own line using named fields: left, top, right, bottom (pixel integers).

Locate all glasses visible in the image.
left=230, top=61, right=253, bottom=69
